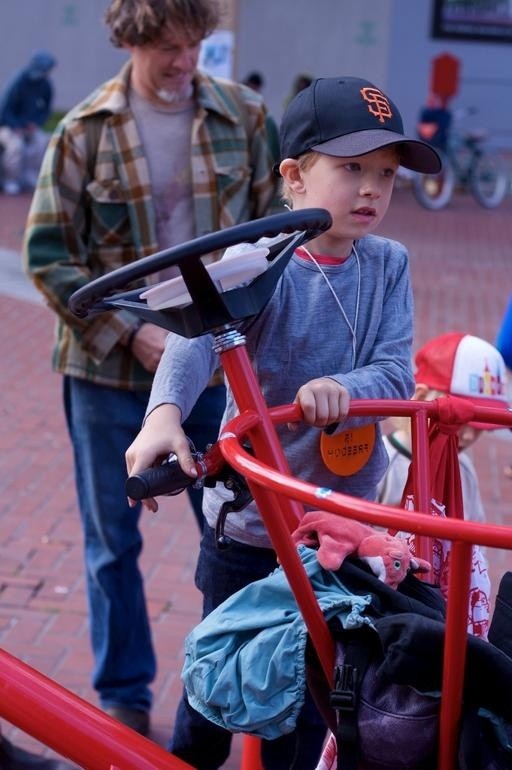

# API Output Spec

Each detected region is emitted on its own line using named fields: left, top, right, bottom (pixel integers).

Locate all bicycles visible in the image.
left=411, top=107, right=507, bottom=211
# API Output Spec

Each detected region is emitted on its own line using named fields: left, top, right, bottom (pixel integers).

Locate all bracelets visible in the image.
left=125, top=316, right=147, bottom=353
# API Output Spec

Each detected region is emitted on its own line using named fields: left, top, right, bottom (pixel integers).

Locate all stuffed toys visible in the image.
left=275, top=509, right=432, bottom=591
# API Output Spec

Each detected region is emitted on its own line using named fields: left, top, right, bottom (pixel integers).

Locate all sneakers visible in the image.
left=3, top=169, right=36, bottom=194
left=104, top=708, right=149, bottom=734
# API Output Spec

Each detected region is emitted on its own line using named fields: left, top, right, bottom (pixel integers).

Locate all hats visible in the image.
left=281, top=77, right=441, bottom=175
left=416, top=333, right=512, bottom=430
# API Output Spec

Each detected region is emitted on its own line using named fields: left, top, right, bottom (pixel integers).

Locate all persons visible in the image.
left=120, top=70, right=451, bottom=769
left=365, top=327, right=512, bottom=644
left=1, top=51, right=58, bottom=199
left=241, top=72, right=263, bottom=92
left=283, top=74, right=314, bottom=110
left=19, top=2, right=292, bottom=743
left=482, top=290, right=511, bottom=440
